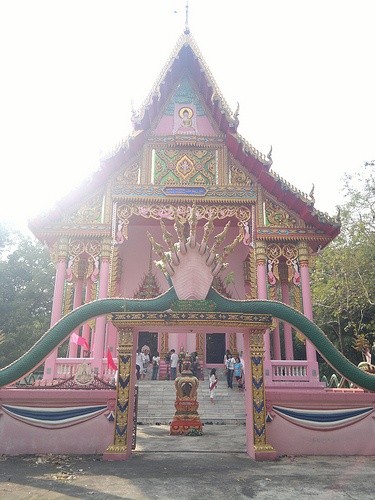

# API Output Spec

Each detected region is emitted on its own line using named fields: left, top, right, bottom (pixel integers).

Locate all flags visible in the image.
left=107, top=349, right=117, bottom=371
left=69, top=333, right=85, bottom=346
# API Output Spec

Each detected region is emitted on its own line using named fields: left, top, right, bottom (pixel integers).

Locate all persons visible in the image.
left=135, top=349, right=178, bottom=380
left=224, top=349, right=229, bottom=369
left=209, top=368, right=217, bottom=403
left=234, top=357, right=243, bottom=389
left=225, top=353, right=236, bottom=389
